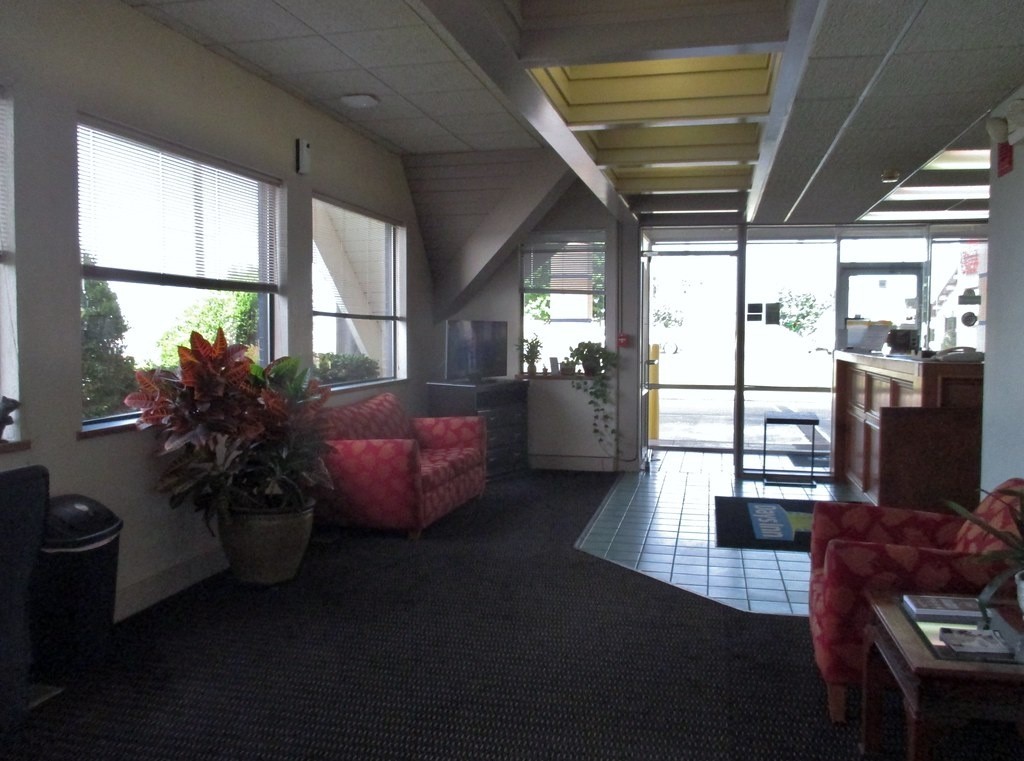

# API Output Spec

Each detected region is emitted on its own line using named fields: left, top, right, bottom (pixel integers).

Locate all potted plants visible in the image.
left=942, top=480, right=1024, bottom=631
left=124, top=325, right=344, bottom=585
left=517, top=332, right=549, bottom=374
left=550, top=341, right=629, bottom=455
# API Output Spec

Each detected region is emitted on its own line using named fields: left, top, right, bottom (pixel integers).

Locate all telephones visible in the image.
left=931, top=345, right=985, bottom=363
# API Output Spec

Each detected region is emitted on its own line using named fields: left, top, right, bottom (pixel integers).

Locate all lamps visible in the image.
left=878, top=174, right=897, bottom=185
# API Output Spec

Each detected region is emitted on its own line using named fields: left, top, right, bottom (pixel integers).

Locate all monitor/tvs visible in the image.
left=445, top=319, right=507, bottom=384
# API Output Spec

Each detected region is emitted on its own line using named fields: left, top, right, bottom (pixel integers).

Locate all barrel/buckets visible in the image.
left=30, top=493, right=123, bottom=680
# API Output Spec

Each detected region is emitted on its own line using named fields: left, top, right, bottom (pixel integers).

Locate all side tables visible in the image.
left=859, top=589, right=1024, bottom=761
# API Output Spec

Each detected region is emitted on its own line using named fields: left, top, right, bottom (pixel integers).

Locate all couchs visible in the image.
left=325, top=393, right=489, bottom=544
left=808, top=477, right=1024, bottom=724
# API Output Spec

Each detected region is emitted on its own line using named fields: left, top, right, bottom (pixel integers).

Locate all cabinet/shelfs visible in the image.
left=426, top=382, right=531, bottom=487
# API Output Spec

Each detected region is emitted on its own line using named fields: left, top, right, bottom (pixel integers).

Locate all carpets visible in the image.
left=715, top=496, right=868, bottom=552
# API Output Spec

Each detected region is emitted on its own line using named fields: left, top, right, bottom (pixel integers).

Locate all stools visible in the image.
left=763, top=410, right=820, bottom=489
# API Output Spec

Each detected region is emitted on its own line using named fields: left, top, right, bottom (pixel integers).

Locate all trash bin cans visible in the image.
left=27, top=493, right=124, bottom=685
